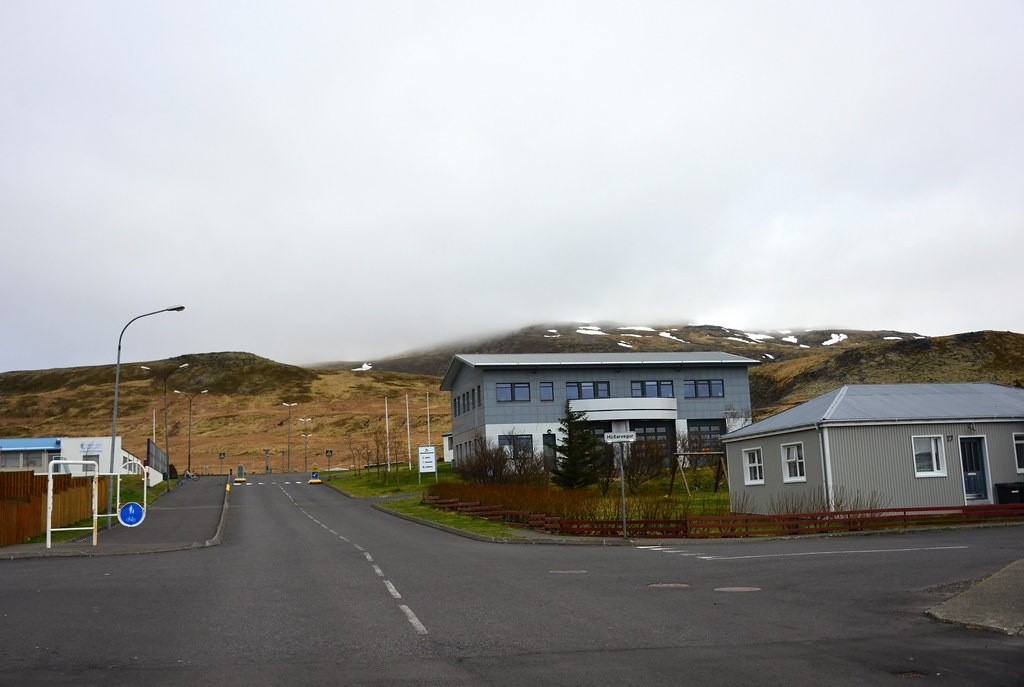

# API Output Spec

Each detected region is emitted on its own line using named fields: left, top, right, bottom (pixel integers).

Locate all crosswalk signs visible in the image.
left=326, top=450, right=333, bottom=456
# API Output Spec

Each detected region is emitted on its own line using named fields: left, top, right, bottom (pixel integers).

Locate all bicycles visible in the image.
left=180, top=469, right=200, bottom=481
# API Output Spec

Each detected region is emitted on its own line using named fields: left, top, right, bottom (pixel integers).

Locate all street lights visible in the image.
left=139, top=363, right=189, bottom=491
left=283, top=402, right=299, bottom=471
left=172, top=391, right=208, bottom=474
left=106, top=306, right=186, bottom=532
left=299, top=417, right=312, bottom=473
